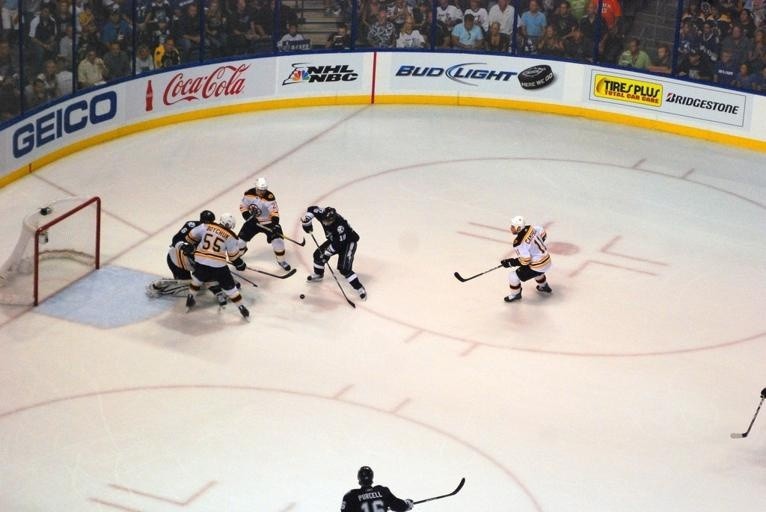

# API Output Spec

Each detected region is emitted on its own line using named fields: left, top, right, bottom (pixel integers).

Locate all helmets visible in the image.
left=321, top=207, right=336, bottom=222
left=220, top=214, right=236, bottom=228
left=511, top=216, right=524, bottom=230
left=357, top=466, right=374, bottom=485
left=200, top=210, right=214, bottom=222
left=255, top=177, right=267, bottom=191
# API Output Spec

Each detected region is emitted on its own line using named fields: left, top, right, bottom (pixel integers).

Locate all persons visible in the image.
left=648, top=47, right=673, bottom=74
left=303, top=206, right=366, bottom=299
left=235, top=178, right=291, bottom=272
left=616, top=38, right=650, bottom=70
left=145, top=210, right=249, bottom=317
left=501, top=216, right=553, bottom=301
left=325, top=0, right=623, bottom=63
left=341, top=465, right=412, bottom=511
left=677, top=1, right=766, bottom=92
left=3, top=1, right=311, bottom=124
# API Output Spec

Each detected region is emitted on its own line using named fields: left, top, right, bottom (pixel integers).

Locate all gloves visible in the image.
left=246, top=215, right=260, bottom=229
left=320, top=254, right=329, bottom=263
left=272, top=223, right=280, bottom=232
left=233, top=258, right=245, bottom=271
left=502, top=259, right=515, bottom=267
left=303, top=224, right=313, bottom=232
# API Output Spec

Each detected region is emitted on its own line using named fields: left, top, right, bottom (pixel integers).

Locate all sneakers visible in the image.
left=359, top=287, right=365, bottom=298
left=279, top=262, right=290, bottom=271
left=308, top=274, right=323, bottom=280
left=217, top=294, right=227, bottom=305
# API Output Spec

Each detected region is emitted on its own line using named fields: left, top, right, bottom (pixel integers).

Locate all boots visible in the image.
left=186, top=295, right=194, bottom=306
left=239, top=306, right=249, bottom=317
left=505, top=289, right=522, bottom=301
left=536, top=284, right=552, bottom=292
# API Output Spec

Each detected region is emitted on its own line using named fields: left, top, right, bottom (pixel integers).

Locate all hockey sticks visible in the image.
left=730, top=396, right=765, bottom=438
left=413, top=478, right=464, bottom=504
left=226, top=261, right=297, bottom=278
left=231, top=272, right=257, bottom=288
left=311, top=233, right=355, bottom=308
left=454, top=265, right=502, bottom=282
left=256, top=223, right=305, bottom=246
